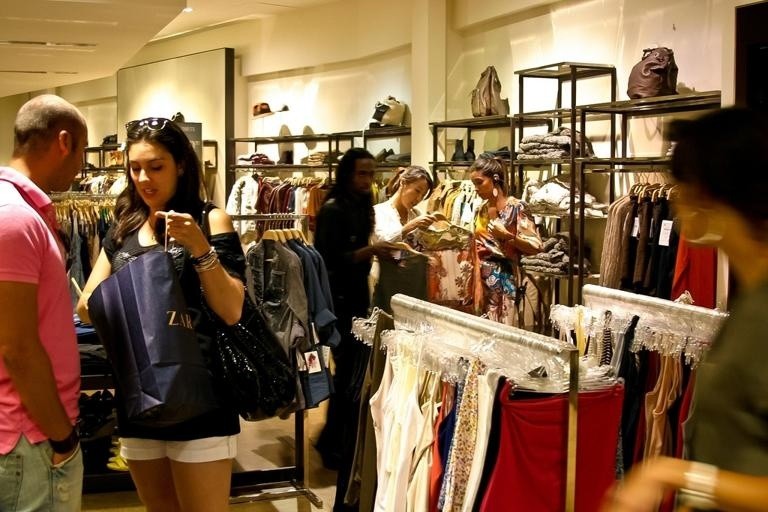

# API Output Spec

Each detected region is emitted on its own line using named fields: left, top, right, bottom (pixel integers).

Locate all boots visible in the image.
left=276, top=150, right=294, bottom=165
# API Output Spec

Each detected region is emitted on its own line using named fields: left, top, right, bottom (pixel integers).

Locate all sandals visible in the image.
left=450, top=138, right=466, bottom=162
left=76, top=389, right=131, bottom=474
left=464, top=138, right=477, bottom=162
left=249, top=153, right=275, bottom=166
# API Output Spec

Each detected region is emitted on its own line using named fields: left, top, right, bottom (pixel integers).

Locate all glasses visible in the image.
left=124, top=117, right=175, bottom=134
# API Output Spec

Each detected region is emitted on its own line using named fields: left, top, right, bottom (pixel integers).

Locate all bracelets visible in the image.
left=48, top=424, right=79, bottom=454
left=678, top=461, right=718, bottom=511
left=194, top=245, right=220, bottom=272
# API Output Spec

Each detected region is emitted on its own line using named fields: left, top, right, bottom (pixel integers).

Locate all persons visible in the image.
left=370, top=166, right=439, bottom=317
left=313, top=148, right=394, bottom=471
left=77, top=117, right=249, bottom=512
left=609, top=104, right=768, bottom=512
left=0, top=93, right=92, bottom=511
left=470, top=153, right=541, bottom=329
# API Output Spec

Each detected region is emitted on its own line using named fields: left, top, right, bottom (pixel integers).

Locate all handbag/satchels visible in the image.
left=367, top=94, right=409, bottom=130
left=193, top=197, right=302, bottom=425
left=623, top=46, right=682, bottom=101
left=63, top=209, right=242, bottom=443
left=464, top=62, right=513, bottom=119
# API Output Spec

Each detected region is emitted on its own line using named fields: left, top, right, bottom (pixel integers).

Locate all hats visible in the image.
left=249, top=101, right=275, bottom=121
